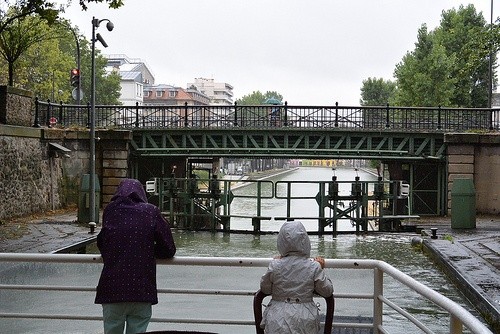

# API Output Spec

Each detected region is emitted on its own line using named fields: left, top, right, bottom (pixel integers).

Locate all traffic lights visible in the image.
left=71, top=68, right=79, bottom=87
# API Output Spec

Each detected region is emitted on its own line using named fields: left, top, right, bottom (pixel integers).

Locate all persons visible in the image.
left=94, top=179, right=177, bottom=334
left=259, top=221, right=334, bottom=334
left=270, top=104, right=281, bottom=127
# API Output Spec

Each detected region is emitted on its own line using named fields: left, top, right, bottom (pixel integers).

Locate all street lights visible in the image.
left=89, top=16, right=114, bottom=232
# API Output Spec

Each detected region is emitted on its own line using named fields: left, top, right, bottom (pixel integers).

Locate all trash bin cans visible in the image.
left=450, top=178, right=476, bottom=229
left=78, top=174, right=100, bottom=225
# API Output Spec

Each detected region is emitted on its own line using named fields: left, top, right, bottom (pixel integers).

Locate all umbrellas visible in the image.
left=262, top=98, right=282, bottom=112
